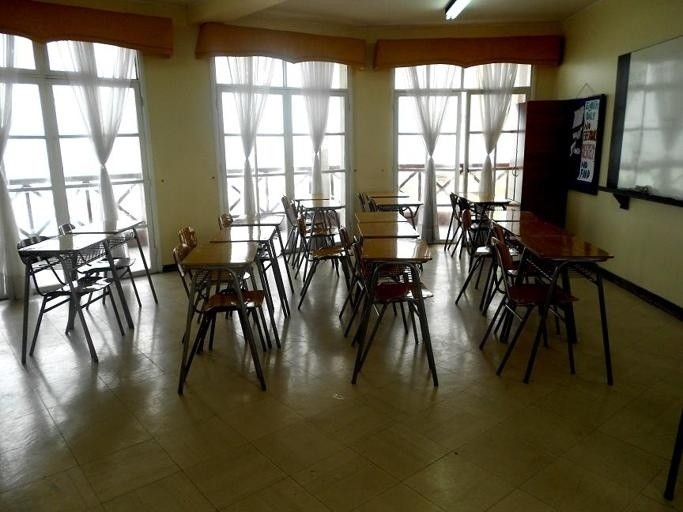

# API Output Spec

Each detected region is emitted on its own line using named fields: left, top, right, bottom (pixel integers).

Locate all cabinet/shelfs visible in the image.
left=512, top=100, right=572, bottom=227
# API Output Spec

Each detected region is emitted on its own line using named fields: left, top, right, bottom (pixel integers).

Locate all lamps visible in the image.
left=445, top=0, right=471, bottom=20
